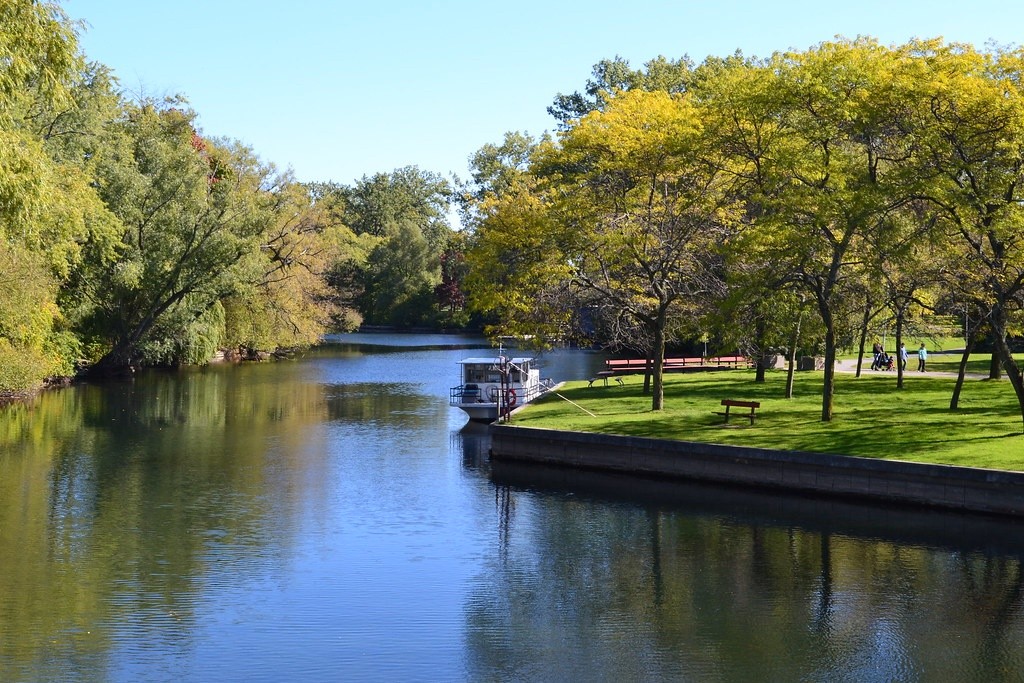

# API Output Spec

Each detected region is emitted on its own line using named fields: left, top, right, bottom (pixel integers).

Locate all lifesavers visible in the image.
left=505, top=389, right=516, bottom=406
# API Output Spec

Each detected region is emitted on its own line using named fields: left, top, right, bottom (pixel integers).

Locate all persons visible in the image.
left=900, top=343, right=908, bottom=371
left=917, top=343, right=927, bottom=373
left=871, top=338, right=884, bottom=371
left=888, top=356, right=893, bottom=365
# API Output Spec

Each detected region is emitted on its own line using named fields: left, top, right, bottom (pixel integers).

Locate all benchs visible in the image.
left=615, top=377, right=624, bottom=387
left=588, top=378, right=597, bottom=388
left=712, top=399, right=761, bottom=425
left=607, top=358, right=756, bottom=374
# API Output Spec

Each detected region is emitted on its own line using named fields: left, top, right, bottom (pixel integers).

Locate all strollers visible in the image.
left=879, top=352, right=896, bottom=371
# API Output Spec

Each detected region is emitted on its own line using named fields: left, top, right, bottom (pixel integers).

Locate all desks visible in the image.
left=596, top=371, right=615, bottom=388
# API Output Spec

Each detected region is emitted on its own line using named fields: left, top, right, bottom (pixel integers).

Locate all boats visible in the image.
left=449, top=334, right=556, bottom=425
